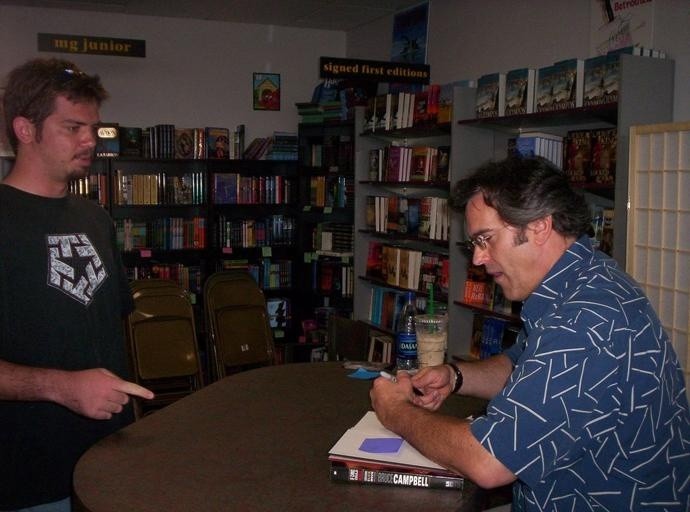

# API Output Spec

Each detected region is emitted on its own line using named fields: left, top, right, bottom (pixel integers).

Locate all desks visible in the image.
left=63, top=363, right=492, bottom=510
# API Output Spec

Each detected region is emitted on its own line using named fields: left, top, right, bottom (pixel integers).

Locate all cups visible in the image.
left=415, top=313, right=447, bottom=372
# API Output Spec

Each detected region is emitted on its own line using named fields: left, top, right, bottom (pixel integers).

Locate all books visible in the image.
left=329, top=411, right=475, bottom=490
left=293, top=99, right=355, bottom=328
left=110, top=210, right=295, bottom=251
left=368, top=285, right=429, bottom=362
left=367, top=195, right=452, bottom=297
left=366, top=79, right=475, bottom=186
left=465, top=263, right=527, bottom=361
left=92, top=122, right=298, bottom=160
left=508, top=129, right=619, bottom=256
left=474, top=46, right=667, bottom=118
left=127, top=261, right=290, bottom=291
left=69, top=168, right=306, bottom=205
left=265, top=298, right=287, bottom=328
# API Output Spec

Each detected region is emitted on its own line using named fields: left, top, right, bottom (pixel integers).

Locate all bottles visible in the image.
left=397, top=290, right=419, bottom=372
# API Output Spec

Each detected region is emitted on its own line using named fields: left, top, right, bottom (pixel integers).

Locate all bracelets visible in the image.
left=445, top=362, right=464, bottom=394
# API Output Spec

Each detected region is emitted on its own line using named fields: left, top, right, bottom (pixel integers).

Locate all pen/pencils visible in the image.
left=380, top=370, right=426, bottom=396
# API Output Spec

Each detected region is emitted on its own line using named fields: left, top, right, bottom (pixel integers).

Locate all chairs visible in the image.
left=124, top=271, right=278, bottom=419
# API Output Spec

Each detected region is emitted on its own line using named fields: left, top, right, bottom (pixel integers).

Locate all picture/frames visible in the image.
left=253, top=72, right=281, bottom=111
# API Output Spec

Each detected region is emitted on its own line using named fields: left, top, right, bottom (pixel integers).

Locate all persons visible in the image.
left=366, top=154, right=689, bottom=512
left=0, top=58, right=156, bottom=511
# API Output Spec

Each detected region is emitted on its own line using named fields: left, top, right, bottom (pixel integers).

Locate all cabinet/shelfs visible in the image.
left=111, top=159, right=299, bottom=346
left=448, top=53, right=675, bottom=360
left=1, top=156, right=110, bottom=211
left=299, top=121, right=353, bottom=361
left=354, top=87, right=453, bottom=362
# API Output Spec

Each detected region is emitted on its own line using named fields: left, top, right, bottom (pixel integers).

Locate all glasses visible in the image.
left=466, top=226, right=505, bottom=249
